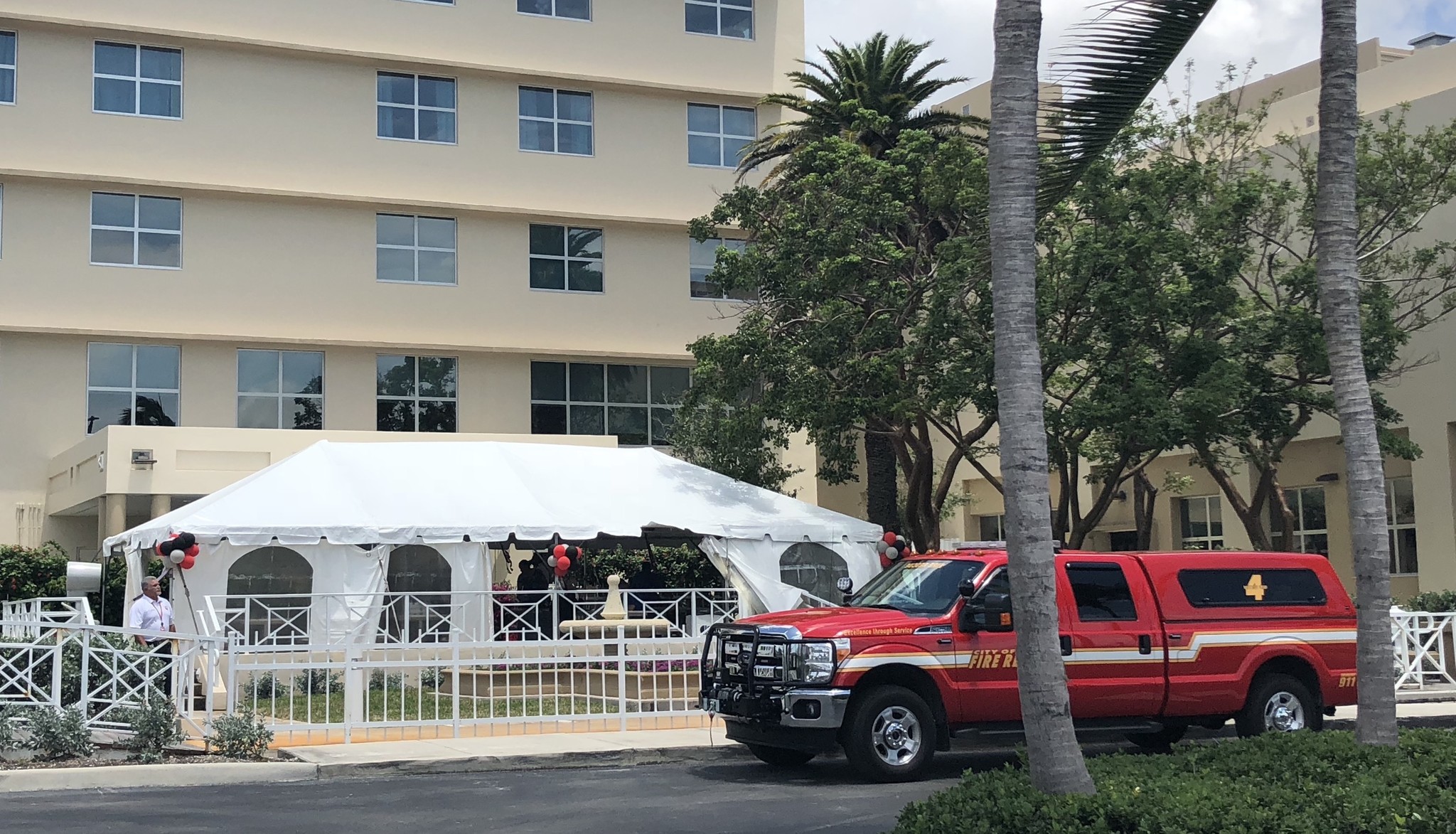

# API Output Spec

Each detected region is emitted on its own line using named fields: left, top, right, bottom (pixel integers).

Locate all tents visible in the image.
left=101, top=440, right=883, bottom=670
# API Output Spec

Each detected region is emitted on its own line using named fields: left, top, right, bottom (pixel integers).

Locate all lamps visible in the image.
left=1315, top=473, right=1338, bottom=482
left=132, top=452, right=157, bottom=465
left=1113, top=490, right=1126, bottom=500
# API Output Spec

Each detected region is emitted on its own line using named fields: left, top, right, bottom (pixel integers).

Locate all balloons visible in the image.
left=547, top=543, right=582, bottom=576
left=877, top=531, right=910, bottom=566
left=157, top=531, right=199, bottom=569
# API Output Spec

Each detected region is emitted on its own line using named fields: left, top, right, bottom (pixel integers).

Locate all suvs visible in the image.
left=698, top=549, right=1356, bottom=781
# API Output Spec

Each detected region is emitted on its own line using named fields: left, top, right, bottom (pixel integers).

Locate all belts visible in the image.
left=147, top=639, right=166, bottom=647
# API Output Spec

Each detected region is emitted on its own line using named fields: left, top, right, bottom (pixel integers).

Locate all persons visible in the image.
left=129, top=576, right=176, bottom=696
left=517, top=559, right=658, bottom=603
